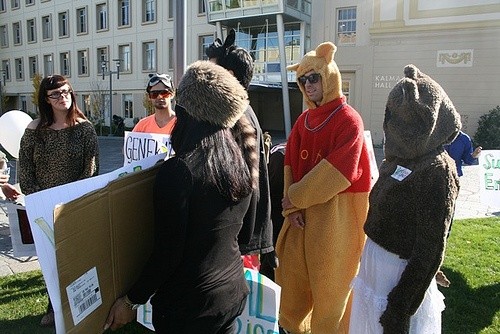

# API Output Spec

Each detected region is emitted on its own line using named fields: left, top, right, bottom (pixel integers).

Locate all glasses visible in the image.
left=299, top=73, right=319, bottom=84
left=49, top=89, right=74, bottom=97
left=148, top=88, right=169, bottom=98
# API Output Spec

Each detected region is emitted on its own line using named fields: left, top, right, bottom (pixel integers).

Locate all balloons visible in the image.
left=0, top=109, right=34, bottom=159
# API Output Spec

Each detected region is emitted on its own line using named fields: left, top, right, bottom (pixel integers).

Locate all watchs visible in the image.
left=122, top=295, right=141, bottom=311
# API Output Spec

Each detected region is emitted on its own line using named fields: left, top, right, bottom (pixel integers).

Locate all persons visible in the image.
left=0, top=150, right=21, bottom=201
left=259, top=132, right=290, bottom=334
left=112, top=115, right=126, bottom=137
left=18, top=74, right=100, bottom=326
left=275, top=41, right=378, bottom=334
left=104, top=58, right=259, bottom=334
left=443, top=131, right=482, bottom=177
left=346, top=64, right=460, bottom=334
left=121, top=72, right=179, bottom=157
left=205, top=28, right=274, bottom=273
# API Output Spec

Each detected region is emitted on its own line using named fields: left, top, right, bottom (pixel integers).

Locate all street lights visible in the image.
left=0, top=70, right=7, bottom=115
left=98, top=59, right=124, bottom=136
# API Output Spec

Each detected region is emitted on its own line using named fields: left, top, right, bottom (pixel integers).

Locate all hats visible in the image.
left=145, top=73, right=172, bottom=88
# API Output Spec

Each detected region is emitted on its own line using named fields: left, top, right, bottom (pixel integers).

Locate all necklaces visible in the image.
left=303, top=104, right=348, bottom=133
